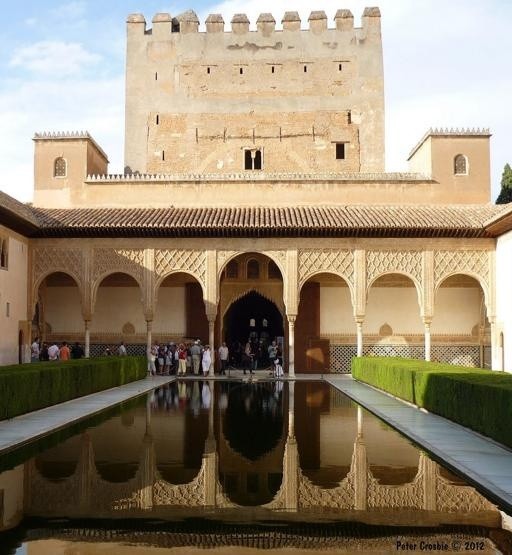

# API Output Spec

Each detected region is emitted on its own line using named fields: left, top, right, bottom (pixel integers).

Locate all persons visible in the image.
left=104, top=347, right=113, bottom=356
left=149, top=336, right=285, bottom=378
left=148, top=379, right=285, bottom=419
left=30, top=336, right=85, bottom=362
left=119, top=341, right=127, bottom=355
